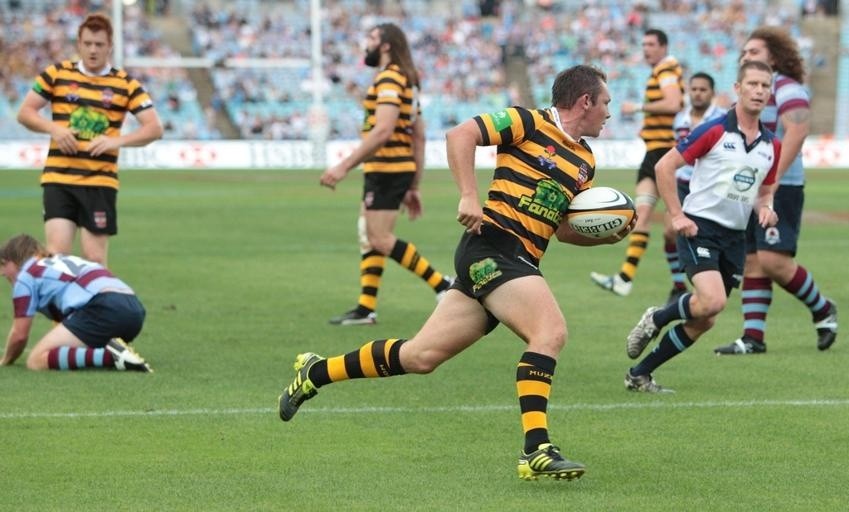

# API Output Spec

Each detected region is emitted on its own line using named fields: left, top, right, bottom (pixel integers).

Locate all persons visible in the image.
left=672, top=71, right=741, bottom=297
left=589, top=28, right=687, bottom=301
left=714, top=26, right=839, bottom=355
left=623, top=61, right=782, bottom=396
left=16, top=13, right=164, bottom=269
left=318, top=23, right=456, bottom=326
left=278, top=67, right=639, bottom=481
left=0, top=0, right=808, bottom=142
left=0, top=234, right=154, bottom=373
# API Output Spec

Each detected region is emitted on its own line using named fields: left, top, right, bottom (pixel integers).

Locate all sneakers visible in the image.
left=626, top=369, right=673, bottom=395
left=329, top=309, right=376, bottom=326
left=716, top=336, right=766, bottom=355
left=106, top=336, right=152, bottom=371
left=816, top=304, right=838, bottom=351
left=627, top=306, right=664, bottom=361
left=278, top=352, right=326, bottom=423
left=518, top=442, right=583, bottom=480
left=592, top=271, right=632, bottom=296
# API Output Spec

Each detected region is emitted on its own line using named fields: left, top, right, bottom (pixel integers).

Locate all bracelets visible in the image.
left=763, top=204, right=773, bottom=211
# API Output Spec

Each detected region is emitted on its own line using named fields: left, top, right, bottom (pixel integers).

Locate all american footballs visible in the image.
left=568, top=187, right=635, bottom=239
